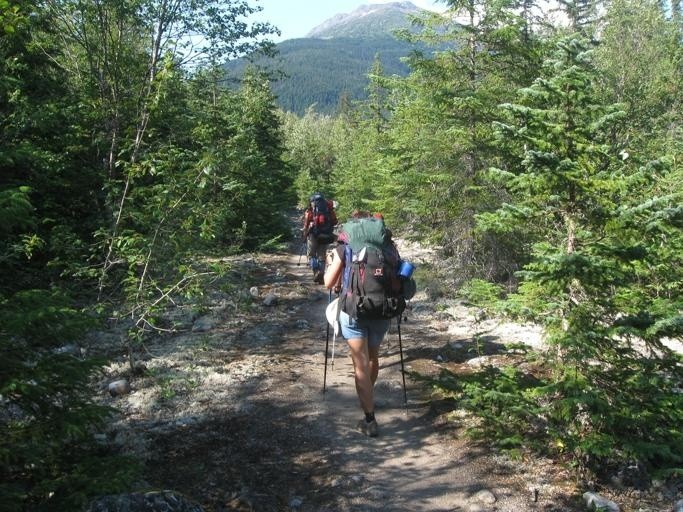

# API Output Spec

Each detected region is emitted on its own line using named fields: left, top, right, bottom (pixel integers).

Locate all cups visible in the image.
left=397, top=261, right=416, bottom=280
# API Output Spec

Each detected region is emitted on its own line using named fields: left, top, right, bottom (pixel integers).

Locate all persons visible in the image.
left=323, top=209, right=416, bottom=437
left=302, top=191, right=338, bottom=285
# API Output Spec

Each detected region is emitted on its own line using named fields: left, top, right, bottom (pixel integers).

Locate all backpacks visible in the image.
left=337, top=218, right=406, bottom=325
left=308, top=198, right=338, bottom=243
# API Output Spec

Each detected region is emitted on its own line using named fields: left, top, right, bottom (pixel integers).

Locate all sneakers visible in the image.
left=362, top=418, right=378, bottom=436
left=313, top=269, right=324, bottom=284
